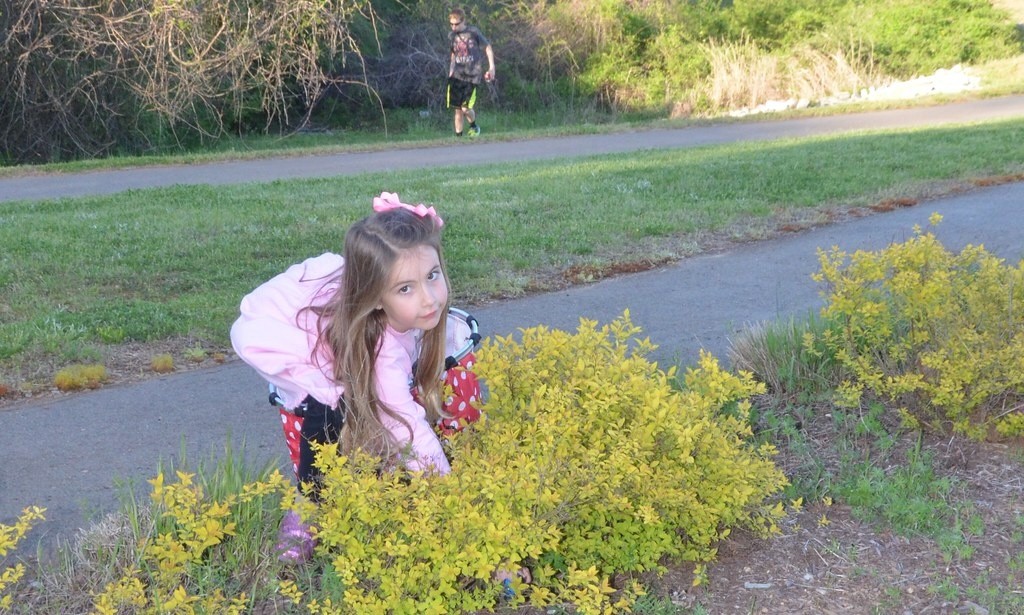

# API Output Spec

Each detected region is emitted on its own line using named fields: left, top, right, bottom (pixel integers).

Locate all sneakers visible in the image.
left=467, top=125, right=480, bottom=137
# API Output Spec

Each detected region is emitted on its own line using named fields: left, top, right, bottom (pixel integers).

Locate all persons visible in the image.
left=447, top=9, right=495, bottom=138
left=229, top=191, right=533, bottom=598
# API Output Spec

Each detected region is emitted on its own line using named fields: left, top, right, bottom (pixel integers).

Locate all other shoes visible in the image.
left=277, top=509, right=318, bottom=564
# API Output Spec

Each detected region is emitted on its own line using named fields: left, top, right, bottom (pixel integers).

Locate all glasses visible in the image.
left=449, top=21, right=462, bottom=26
